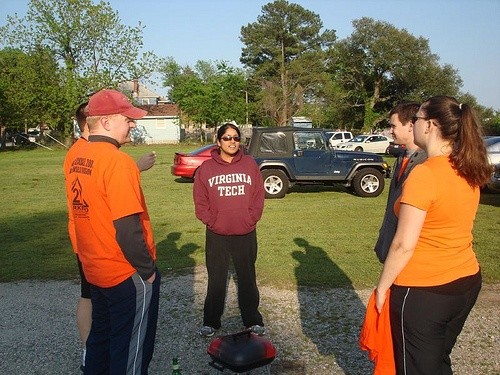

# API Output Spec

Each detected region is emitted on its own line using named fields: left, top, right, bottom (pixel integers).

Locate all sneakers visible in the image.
left=248, top=324, right=265, bottom=336
left=200, top=326, right=218, bottom=337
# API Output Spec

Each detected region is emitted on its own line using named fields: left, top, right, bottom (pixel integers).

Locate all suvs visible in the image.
left=247, top=126, right=388, bottom=199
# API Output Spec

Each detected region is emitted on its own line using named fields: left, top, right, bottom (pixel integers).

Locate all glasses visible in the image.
left=220, top=136, right=241, bottom=142
left=412, top=116, right=438, bottom=127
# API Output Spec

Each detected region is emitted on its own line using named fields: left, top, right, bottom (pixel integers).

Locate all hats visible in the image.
left=87, top=88, right=148, bottom=120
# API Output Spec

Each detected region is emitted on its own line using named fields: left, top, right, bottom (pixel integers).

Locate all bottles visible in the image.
left=171, top=357, right=182, bottom=375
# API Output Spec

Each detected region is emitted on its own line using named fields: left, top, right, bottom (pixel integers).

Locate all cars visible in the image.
left=483, top=136, right=500, bottom=193
left=12, top=134, right=30, bottom=145
left=171, top=144, right=219, bottom=179
left=326, top=132, right=405, bottom=157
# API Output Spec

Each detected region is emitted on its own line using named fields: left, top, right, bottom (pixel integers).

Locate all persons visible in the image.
left=374, top=94, right=492, bottom=375
left=63, top=88, right=161, bottom=375
left=193, top=124, right=266, bottom=337
